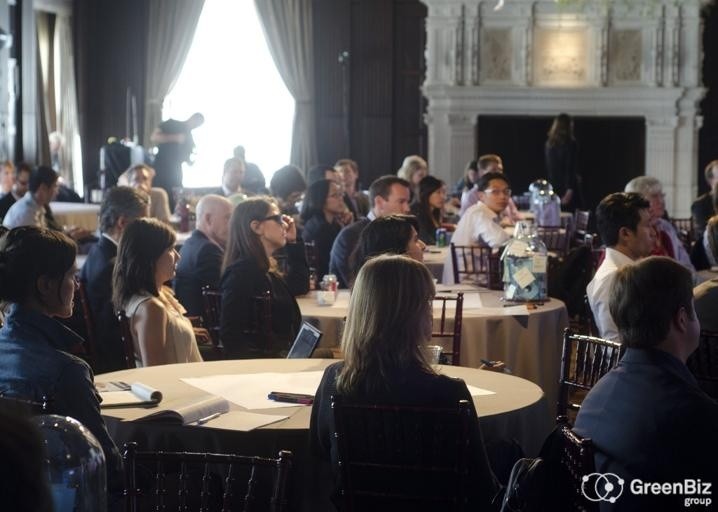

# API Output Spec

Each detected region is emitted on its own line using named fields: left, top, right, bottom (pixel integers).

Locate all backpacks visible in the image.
left=489, top=419, right=578, bottom=512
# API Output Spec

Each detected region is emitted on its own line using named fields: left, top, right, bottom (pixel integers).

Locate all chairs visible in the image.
left=73, top=278, right=94, bottom=346
left=124, top=441, right=295, bottom=512
left=671, top=215, right=694, bottom=244
left=450, top=242, right=509, bottom=290
left=432, top=292, right=465, bottom=366
left=0, top=390, right=55, bottom=512
left=536, top=223, right=569, bottom=258
left=112, top=310, right=138, bottom=370
left=575, top=209, right=591, bottom=240
left=201, top=284, right=274, bottom=358
left=554, top=327, right=626, bottom=428
left=555, top=413, right=599, bottom=511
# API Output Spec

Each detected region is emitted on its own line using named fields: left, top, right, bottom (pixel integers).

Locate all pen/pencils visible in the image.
left=503, top=301, right=544, bottom=310
left=198, top=412, right=223, bottom=423
left=269, top=389, right=317, bottom=405
left=480, top=358, right=494, bottom=367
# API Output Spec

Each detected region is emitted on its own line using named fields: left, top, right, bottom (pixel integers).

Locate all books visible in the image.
left=97, top=381, right=163, bottom=409
left=119, top=396, right=291, bottom=433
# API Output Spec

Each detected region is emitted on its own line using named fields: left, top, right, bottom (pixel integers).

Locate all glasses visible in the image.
left=262, top=215, right=283, bottom=224
left=484, top=188, right=512, bottom=196
left=329, top=191, right=345, bottom=199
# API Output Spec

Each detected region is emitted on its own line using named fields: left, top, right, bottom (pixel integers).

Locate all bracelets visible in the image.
left=285, top=238, right=297, bottom=243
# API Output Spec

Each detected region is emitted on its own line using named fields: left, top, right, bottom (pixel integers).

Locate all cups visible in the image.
left=427, top=345, right=443, bottom=370
left=317, top=291, right=335, bottom=305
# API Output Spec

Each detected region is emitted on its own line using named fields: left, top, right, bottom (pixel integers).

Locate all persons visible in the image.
left=308, top=165, right=355, bottom=229
left=349, top=214, right=507, bottom=374
left=150, top=112, right=206, bottom=215
left=307, top=166, right=341, bottom=181
left=562, top=254, right=717, bottom=511
left=174, top=193, right=236, bottom=317
left=328, top=175, right=411, bottom=291
left=125, top=164, right=156, bottom=196
left=218, top=198, right=303, bottom=359
left=309, top=253, right=483, bottom=512
left=258, top=164, right=309, bottom=242
left=0, top=224, right=128, bottom=512
left=543, top=112, right=584, bottom=216
left=442, top=153, right=534, bottom=285
left=79, top=185, right=209, bottom=366
left=623, top=174, right=706, bottom=290
left=397, top=154, right=428, bottom=199
left=111, top=215, right=205, bottom=370
left=0, top=129, right=93, bottom=243
left=691, top=159, right=718, bottom=272
left=299, top=178, right=343, bottom=274
left=585, top=191, right=717, bottom=371
left=232, top=144, right=271, bottom=196
left=703, top=181, right=718, bottom=272
left=409, top=173, right=446, bottom=246
left=333, top=158, right=371, bottom=218
left=213, top=155, right=258, bottom=205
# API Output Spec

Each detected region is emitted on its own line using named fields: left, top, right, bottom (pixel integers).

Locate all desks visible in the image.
left=93, top=357, right=545, bottom=466
left=296, top=286, right=567, bottom=424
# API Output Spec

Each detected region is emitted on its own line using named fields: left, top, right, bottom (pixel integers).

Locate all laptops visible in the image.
left=284, top=320, right=324, bottom=358
